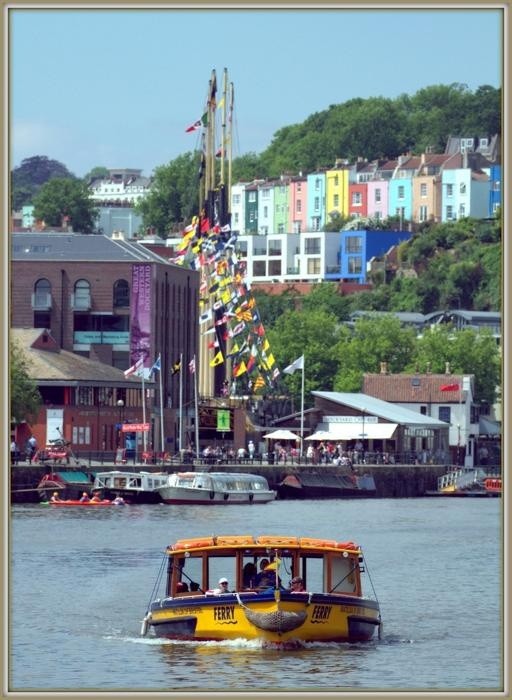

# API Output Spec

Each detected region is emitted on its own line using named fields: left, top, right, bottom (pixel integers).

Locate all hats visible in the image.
left=82, top=491, right=101, bottom=498
left=218, top=578, right=229, bottom=585
left=52, top=491, right=59, bottom=497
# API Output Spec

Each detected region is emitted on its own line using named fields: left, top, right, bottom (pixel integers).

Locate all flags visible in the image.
left=124, top=358, right=142, bottom=380
left=198, top=151, right=206, bottom=178
left=169, top=215, right=281, bottom=393
left=185, top=112, right=208, bottom=133
left=145, top=358, right=160, bottom=379
left=441, top=384, right=461, bottom=392
left=283, top=356, right=304, bottom=375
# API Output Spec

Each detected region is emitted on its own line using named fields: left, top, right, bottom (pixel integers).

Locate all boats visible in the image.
left=276, top=470, right=376, bottom=497
left=11, top=472, right=277, bottom=506
left=141, top=535, right=383, bottom=650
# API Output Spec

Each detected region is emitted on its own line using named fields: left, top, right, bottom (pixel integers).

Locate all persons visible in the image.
left=79, top=492, right=89, bottom=503
left=257, top=559, right=282, bottom=587
left=201, top=430, right=363, bottom=469
left=91, top=492, right=102, bottom=502
left=218, top=578, right=229, bottom=594
left=112, top=494, right=124, bottom=502
left=9, top=433, right=39, bottom=466
left=290, top=577, right=306, bottom=593
left=243, top=563, right=258, bottom=588
left=51, top=492, right=64, bottom=504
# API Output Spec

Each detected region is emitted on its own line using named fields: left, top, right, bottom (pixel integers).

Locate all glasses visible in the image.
left=291, top=581, right=300, bottom=584
left=262, top=562, right=269, bottom=565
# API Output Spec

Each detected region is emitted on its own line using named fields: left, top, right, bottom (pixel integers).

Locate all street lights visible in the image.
left=117, top=400, right=124, bottom=423
